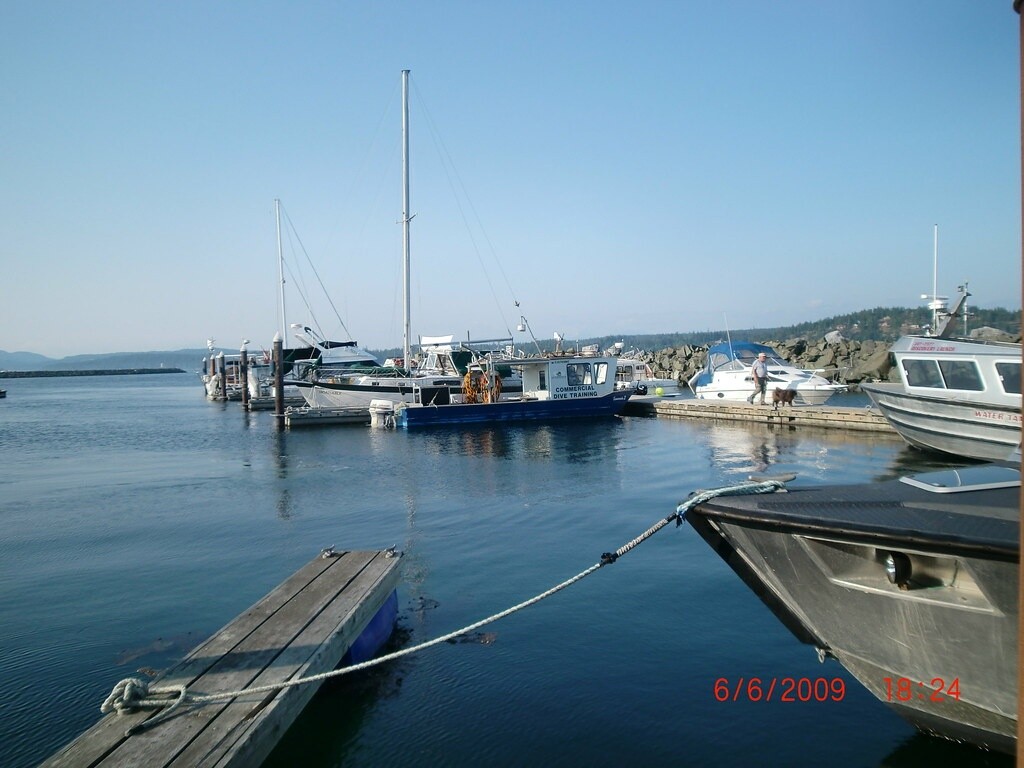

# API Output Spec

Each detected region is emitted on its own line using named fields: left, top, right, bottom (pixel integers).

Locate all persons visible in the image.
left=747, top=353, right=770, bottom=405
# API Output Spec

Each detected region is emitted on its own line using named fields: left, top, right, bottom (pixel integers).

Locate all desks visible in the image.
left=503, top=396, right=538, bottom=400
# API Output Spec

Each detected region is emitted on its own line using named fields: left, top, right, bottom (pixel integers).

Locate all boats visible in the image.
left=581, top=358, right=682, bottom=403
left=367, top=352, right=639, bottom=428
left=859, top=331, right=1022, bottom=470
left=202, top=323, right=383, bottom=400
left=688, top=340, right=851, bottom=405
left=682, top=462, right=1019, bottom=754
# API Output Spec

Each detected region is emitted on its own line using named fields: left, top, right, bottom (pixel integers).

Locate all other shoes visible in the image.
left=747, top=397, right=753, bottom=404
left=761, top=401, right=768, bottom=405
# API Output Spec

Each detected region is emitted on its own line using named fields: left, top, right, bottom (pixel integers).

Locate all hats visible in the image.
left=758, top=353, right=766, bottom=356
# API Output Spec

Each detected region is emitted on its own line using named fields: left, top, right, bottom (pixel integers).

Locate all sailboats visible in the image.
left=297, top=67, right=578, bottom=409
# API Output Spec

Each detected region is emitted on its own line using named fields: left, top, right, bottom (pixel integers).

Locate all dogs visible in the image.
left=772, top=387, right=797, bottom=408
